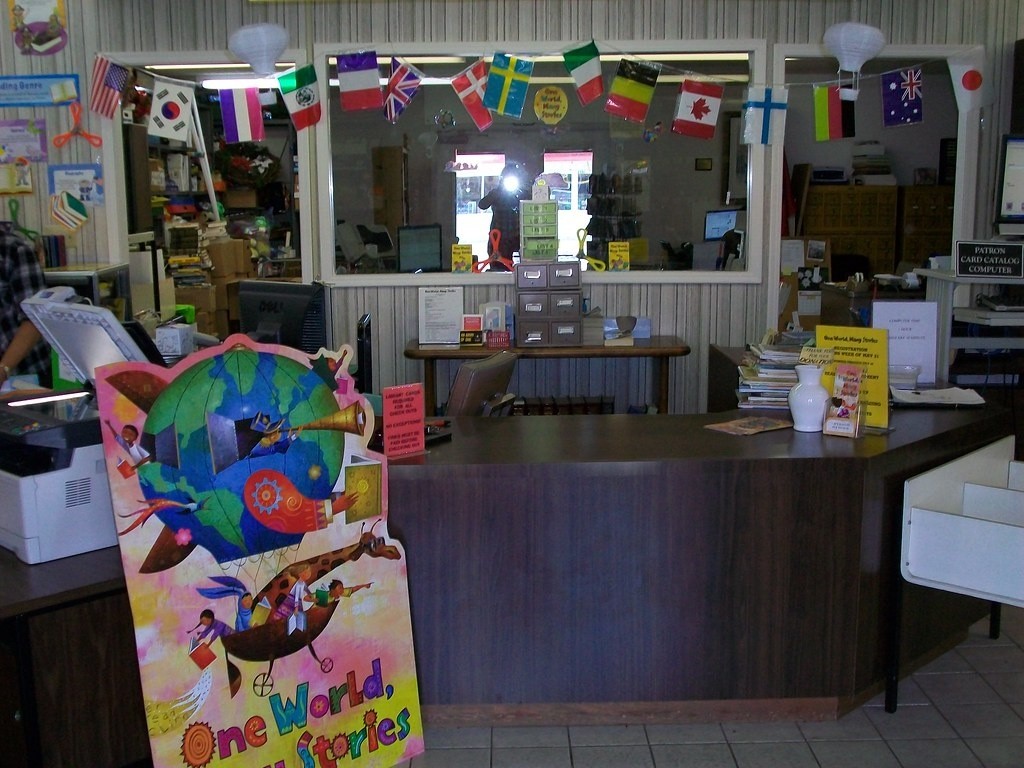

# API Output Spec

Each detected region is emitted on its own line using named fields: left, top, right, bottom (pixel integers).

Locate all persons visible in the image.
left=478, top=164, right=531, bottom=274
left=0, top=229, right=49, bottom=387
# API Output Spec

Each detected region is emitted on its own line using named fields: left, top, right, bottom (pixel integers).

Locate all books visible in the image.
left=736, top=343, right=801, bottom=409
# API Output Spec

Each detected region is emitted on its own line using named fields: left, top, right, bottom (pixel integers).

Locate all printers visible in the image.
left=338, top=221, right=397, bottom=272
left=0, top=286, right=165, bottom=565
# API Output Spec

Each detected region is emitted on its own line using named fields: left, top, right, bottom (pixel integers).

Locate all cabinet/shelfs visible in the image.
left=126, top=126, right=213, bottom=242
left=802, top=184, right=954, bottom=277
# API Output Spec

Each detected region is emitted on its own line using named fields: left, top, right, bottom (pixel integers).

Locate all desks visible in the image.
left=403, top=333, right=690, bottom=416
left=40, top=262, right=133, bottom=323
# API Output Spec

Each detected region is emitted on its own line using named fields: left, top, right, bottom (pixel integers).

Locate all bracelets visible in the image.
left=2, top=362, right=16, bottom=381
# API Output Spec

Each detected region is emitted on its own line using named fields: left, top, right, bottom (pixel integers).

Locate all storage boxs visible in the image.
left=185, top=238, right=259, bottom=337
left=224, top=187, right=258, bottom=208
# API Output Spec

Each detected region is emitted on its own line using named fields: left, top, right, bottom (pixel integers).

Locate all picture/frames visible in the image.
left=719, top=110, right=747, bottom=205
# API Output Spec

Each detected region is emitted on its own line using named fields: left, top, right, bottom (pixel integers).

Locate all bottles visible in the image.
left=788, top=364, right=831, bottom=433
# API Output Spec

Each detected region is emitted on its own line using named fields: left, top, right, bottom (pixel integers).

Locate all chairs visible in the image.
left=444, top=348, right=517, bottom=418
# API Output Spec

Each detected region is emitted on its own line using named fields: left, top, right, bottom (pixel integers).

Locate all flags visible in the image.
left=812, top=85, right=858, bottom=143
left=275, top=63, right=322, bottom=131
left=880, top=64, right=924, bottom=130
left=673, top=80, right=724, bottom=142
left=449, top=52, right=534, bottom=134
left=218, top=88, right=265, bottom=143
left=87, top=55, right=129, bottom=118
left=743, top=86, right=789, bottom=145
left=562, top=42, right=662, bottom=125
left=946, top=44, right=1000, bottom=115
left=335, top=51, right=426, bottom=126
left=145, top=78, right=195, bottom=143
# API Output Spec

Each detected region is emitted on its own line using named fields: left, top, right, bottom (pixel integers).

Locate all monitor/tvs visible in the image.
left=397, top=224, right=443, bottom=271
left=704, top=209, right=738, bottom=245
left=42, top=270, right=100, bottom=306
left=226, top=280, right=333, bottom=354
left=995, top=134, right=1024, bottom=236
